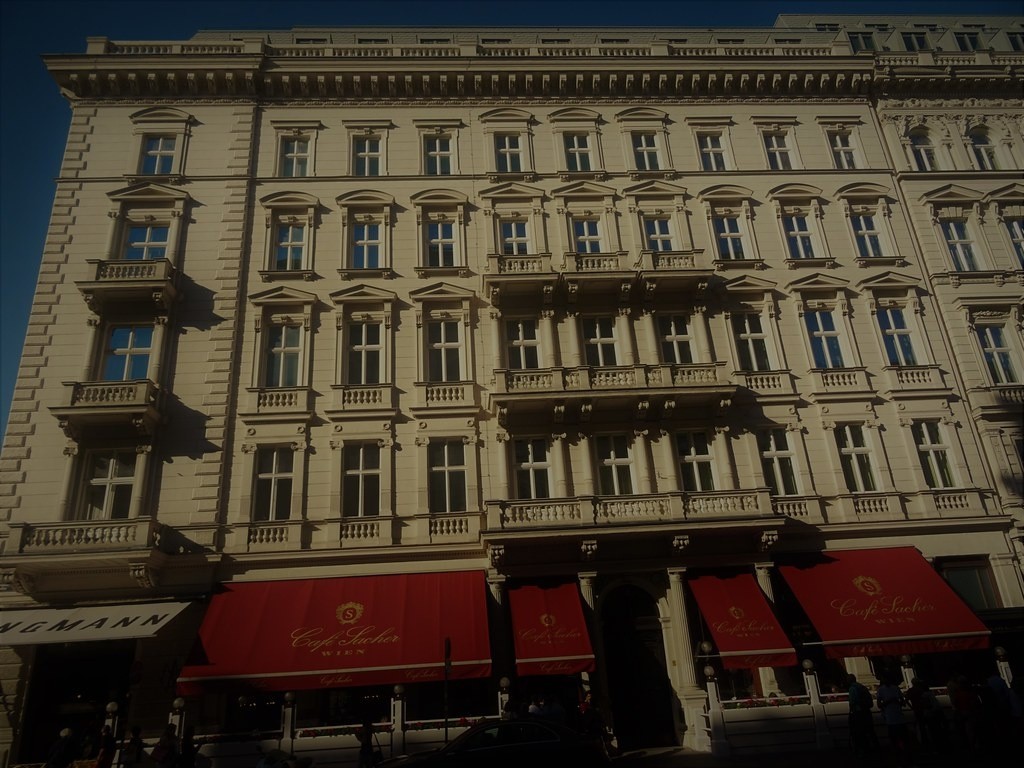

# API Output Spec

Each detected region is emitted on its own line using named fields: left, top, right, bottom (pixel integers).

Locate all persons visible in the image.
left=502, top=693, right=543, bottom=718
left=43, top=724, right=320, bottom=767
left=845, top=666, right=1022, bottom=762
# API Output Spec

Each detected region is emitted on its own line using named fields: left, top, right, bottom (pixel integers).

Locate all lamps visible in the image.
left=284, top=692, right=295, bottom=708
left=394, top=684, right=405, bottom=701
left=703, top=665, right=716, bottom=682
left=499, top=677, right=511, bottom=695
left=901, top=655, right=912, bottom=668
left=701, top=642, right=712, bottom=654
left=994, top=646, right=1007, bottom=662
left=172, top=697, right=184, bottom=716
left=802, top=659, right=814, bottom=675
left=106, top=701, right=118, bottom=719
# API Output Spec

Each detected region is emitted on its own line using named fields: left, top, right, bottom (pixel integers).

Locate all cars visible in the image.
left=373, top=716, right=602, bottom=768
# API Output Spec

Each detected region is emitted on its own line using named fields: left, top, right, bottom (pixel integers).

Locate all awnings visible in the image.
left=0, top=594, right=196, bottom=648
left=506, top=583, right=597, bottom=678
left=689, top=569, right=801, bottom=670
left=774, top=547, right=992, bottom=662
left=174, top=570, right=494, bottom=694
